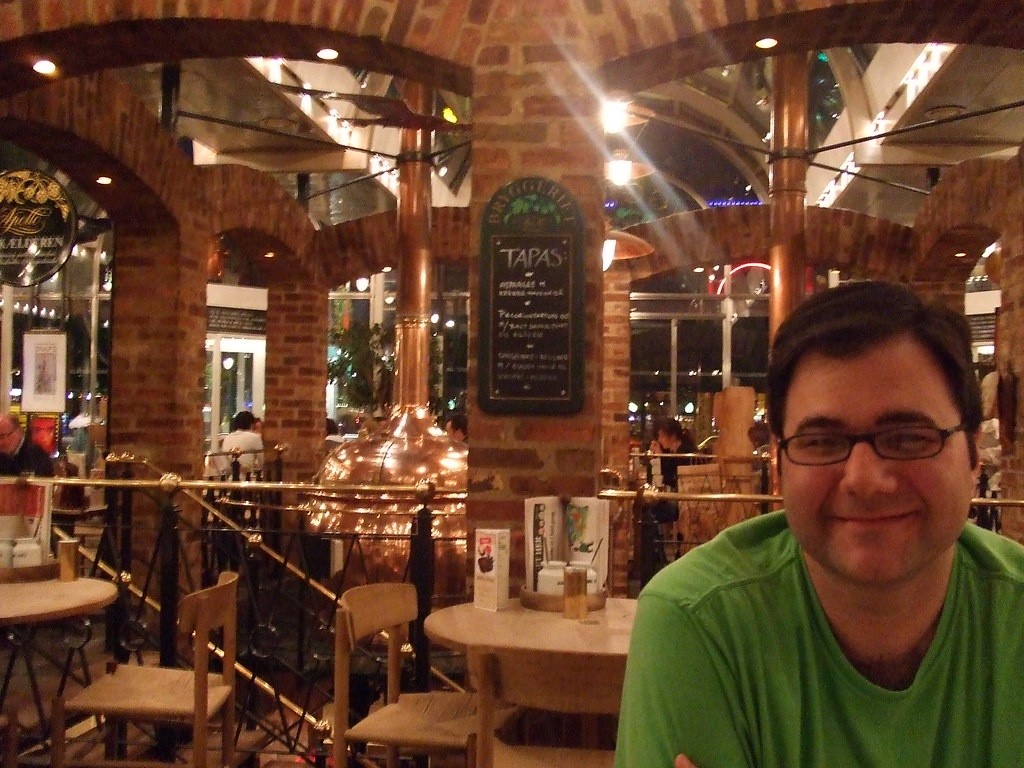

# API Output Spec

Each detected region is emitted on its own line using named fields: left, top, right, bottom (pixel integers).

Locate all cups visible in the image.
left=563, top=567, right=588, bottom=619
left=59, top=540, right=80, bottom=581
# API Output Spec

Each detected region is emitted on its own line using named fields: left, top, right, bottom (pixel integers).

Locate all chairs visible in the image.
left=333, top=583, right=525, bottom=768
left=49, top=572, right=239, bottom=768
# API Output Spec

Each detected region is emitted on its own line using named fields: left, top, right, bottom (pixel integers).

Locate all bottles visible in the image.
left=0, top=537, right=14, bottom=568
left=537, top=562, right=566, bottom=595
left=13, top=538, right=42, bottom=568
left=569, top=561, right=597, bottom=594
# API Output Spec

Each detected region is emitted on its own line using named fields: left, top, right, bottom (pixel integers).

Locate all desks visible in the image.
left=0, top=573, right=119, bottom=755
left=424, top=598, right=641, bottom=768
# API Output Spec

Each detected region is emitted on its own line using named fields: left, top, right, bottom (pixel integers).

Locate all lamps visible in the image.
left=604, top=148, right=656, bottom=184
left=603, top=90, right=657, bottom=132
left=602, top=230, right=653, bottom=269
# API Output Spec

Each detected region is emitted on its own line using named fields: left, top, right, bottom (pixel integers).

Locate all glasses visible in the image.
left=780, top=424, right=964, bottom=465
left=0, top=426, right=19, bottom=440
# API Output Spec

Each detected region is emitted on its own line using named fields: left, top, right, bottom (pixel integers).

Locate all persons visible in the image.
left=614, top=281, right=1024, bottom=767
left=641, top=416, right=698, bottom=564
left=0, top=410, right=53, bottom=479
left=446, top=415, right=468, bottom=443
left=326, top=414, right=338, bottom=436
left=221, top=411, right=263, bottom=475
